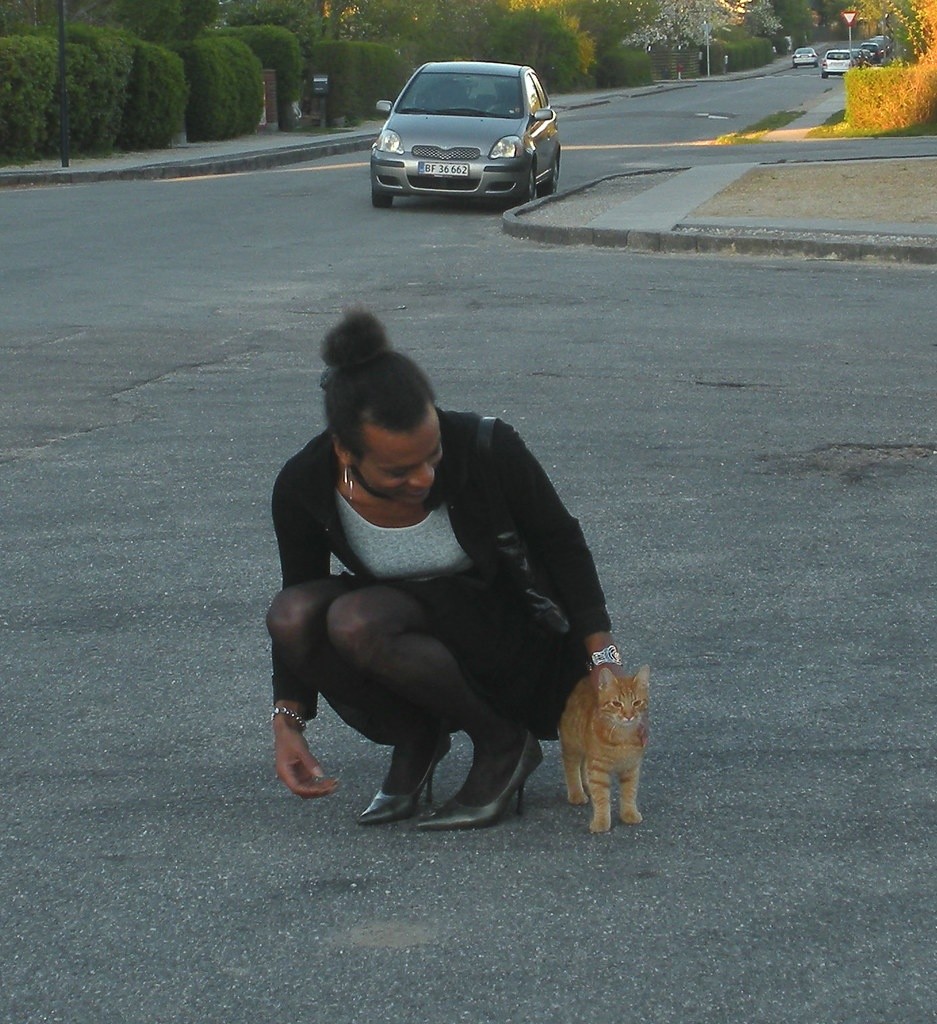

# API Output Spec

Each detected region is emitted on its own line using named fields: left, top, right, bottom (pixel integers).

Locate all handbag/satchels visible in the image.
left=494, top=528, right=574, bottom=654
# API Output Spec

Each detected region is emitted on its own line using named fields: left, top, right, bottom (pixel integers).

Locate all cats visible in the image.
left=557, top=662, right=652, bottom=834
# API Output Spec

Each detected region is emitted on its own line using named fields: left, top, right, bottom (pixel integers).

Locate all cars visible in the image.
left=792, top=48, right=819, bottom=68
left=367, top=61, right=561, bottom=206
left=852, top=49, right=873, bottom=66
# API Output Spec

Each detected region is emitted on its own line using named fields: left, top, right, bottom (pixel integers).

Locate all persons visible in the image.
left=265, top=311, right=650, bottom=831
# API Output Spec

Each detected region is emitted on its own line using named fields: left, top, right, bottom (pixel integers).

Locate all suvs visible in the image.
left=821, top=49, right=856, bottom=79
left=860, top=42, right=883, bottom=63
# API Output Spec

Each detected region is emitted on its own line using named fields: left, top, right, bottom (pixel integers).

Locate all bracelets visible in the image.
left=271, top=707, right=307, bottom=731
left=585, top=644, right=624, bottom=675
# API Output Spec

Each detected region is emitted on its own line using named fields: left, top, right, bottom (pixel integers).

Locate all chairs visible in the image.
left=495, top=81, right=520, bottom=103
left=476, top=94, right=494, bottom=110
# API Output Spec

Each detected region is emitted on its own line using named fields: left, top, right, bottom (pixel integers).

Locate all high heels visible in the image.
left=422, top=727, right=542, bottom=834
left=354, top=735, right=449, bottom=825
